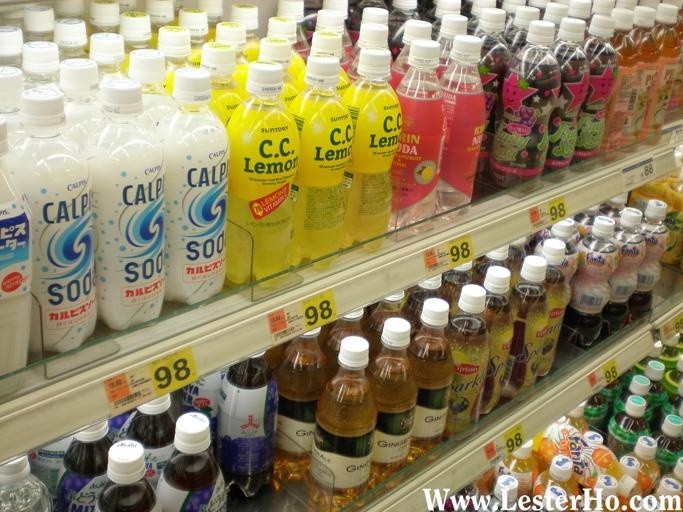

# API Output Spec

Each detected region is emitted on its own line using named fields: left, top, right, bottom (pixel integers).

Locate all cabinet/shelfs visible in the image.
left=1, top=122, right=683, bottom=511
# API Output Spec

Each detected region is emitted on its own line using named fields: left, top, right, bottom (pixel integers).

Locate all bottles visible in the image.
left=628, top=198, right=669, bottom=321
left=480, top=266, right=515, bottom=415
left=570, top=326, right=683, bottom=413
left=491, top=438, right=539, bottom=501
left=2, top=87, right=96, bottom=354
left=570, top=13, right=620, bottom=174
left=488, top=19, right=562, bottom=192
left=390, top=38, right=445, bottom=235
left=563, top=215, right=622, bottom=350
left=541, top=414, right=683, bottom=512
left=537, top=237, right=571, bottom=384
left=605, top=7, right=639, bottom=168
left=501, top=1, right=571, bottom=52
left=366, top=289, right=405, bottom=365
left=75, top=80, right=167, bottom=331
left=439, top=261, right=473, bottom=311
left=474, top=474, right=524, bottom=512
left=272, top=325, right=330, bottom=491
left=401, top=273, right=443, bottom=344
left=0, top=117, right=35, bottom=380
left=502, top=255, right=550, bottom=403
left=320, top=308, right=365, bottom=383
left=366, top=317, right=418, bottom=503
left=638, top=4, right=681, bottom=146
left=179, top=368, right=229, bottom=441
left=147, top=68, right=228, bottom=306
left=598, top=190, right=629, bottom=215
left=122, top=393, right=177, bottom=490
left=439, top=34, right=487, bottom=222
left=342, top=46, right=402, bottom=254
left=543, top=17, right=591, bottom=185
left=226, top=0, right=347, bottom=107
left=572, top=1, right=683, bottom=18
left=289, top=54, right=355, bottom=273
left=508, top=237, right=529, bottom=290
left=573, top=203, right=608, bottom=242
left=214, top=351, right=279, bottom=497
left=628, top=5, right=661, bottom=155
left=93, top=438, right=161, bottom=511
left=309, top=336, right=378, bottom=512
left=574, top=204, right=599, bottom=235
left=155, top=412, right=228, bottom=511
left=441, top=282, right=489, bottom=442
left=601, top=206, right=647, bottom=342
left=533, top=217, right=581, bottom=285
left=472, top=245, right=510, bottom=287
left=0, top=454, right=53, bottom=512
left=55, top=420, right=114, bottom=512
left=1, top=0, right=225, bottom=116
left=348, top=1, right=498, bottom=74
left=472, top=7, right=513, bottom=198
left=226, top=60, right=302, bottom=285
left=406, top=297, right=453, bottom=474
